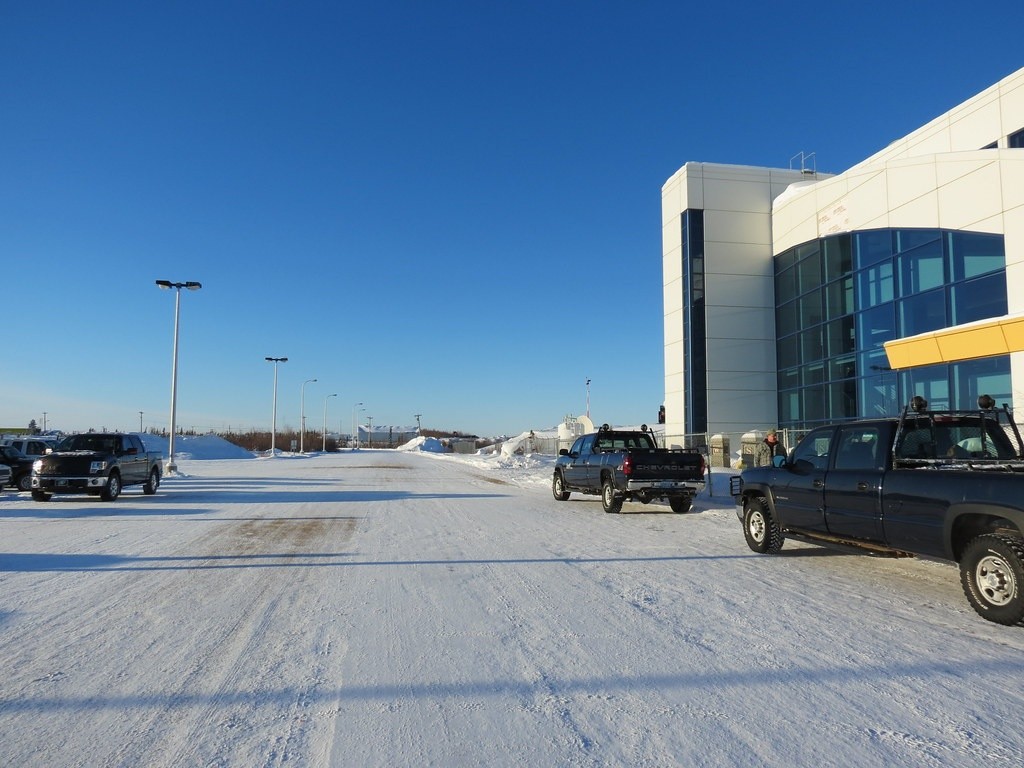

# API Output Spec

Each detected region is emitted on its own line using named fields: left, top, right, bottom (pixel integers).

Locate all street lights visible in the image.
left=368, top=414, right=373, bottom=448
left=300, top=380, right=317, bottom=454
left=155, top=279, right=202, bottom=475
left=139, top=411, right=144, bottom=432
left=264, top=357, right=289, bottom=453
left=357, top=408, right=365, bottom=449
left=351, top=402, right=363, bottom=451
left=322, top=393, right=337, bottom=452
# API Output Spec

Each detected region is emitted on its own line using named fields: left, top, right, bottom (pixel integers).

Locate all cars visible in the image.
left=0, top=430, right=68, bottom=493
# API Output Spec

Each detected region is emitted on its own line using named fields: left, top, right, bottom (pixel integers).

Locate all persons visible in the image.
left=753, top=428, right=787, bottom=468
left=789, top=435, right=818, bottom=457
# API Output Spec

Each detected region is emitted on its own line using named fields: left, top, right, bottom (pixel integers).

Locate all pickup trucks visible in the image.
left=552, top=423, right=706, bottom=513
left=735, top=394, right=1024, bottom=627
left=31, top=432, right=163, bottom=502
